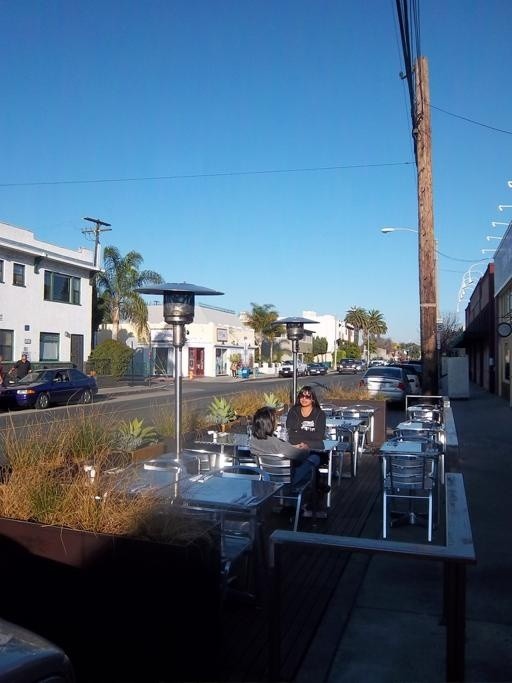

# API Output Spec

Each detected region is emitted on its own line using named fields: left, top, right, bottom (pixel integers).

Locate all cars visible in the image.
left=279, top=359, right=307, bottom=377
left=0, top=367, right=98, bottom=409
left=337, top=357, right=357, bottom=374
left=358, top=356, right=422, bottom=410
left=307, top=362, right=328, bottom=375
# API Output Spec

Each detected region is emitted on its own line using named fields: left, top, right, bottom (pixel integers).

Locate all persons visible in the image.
left=10, top=354, right=31, bottom=382
left=230, top=361, right=242, bottom=378
left=56, top=373, right=67, bottom=382
left=249, top=407, right=328, bottom=518
left=287, top=386, right=330, bottom=494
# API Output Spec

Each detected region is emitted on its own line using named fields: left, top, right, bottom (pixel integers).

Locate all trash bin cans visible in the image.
left=242, top=368, right=249, bottom=378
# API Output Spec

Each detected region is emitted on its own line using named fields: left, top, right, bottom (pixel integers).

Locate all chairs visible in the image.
left=91, top=404, right=379, bottom=598
left=374, top=404, right=446, bottom=544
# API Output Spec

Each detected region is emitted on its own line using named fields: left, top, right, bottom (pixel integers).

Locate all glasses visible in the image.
left=299, top=393, right=313, bottom=400
